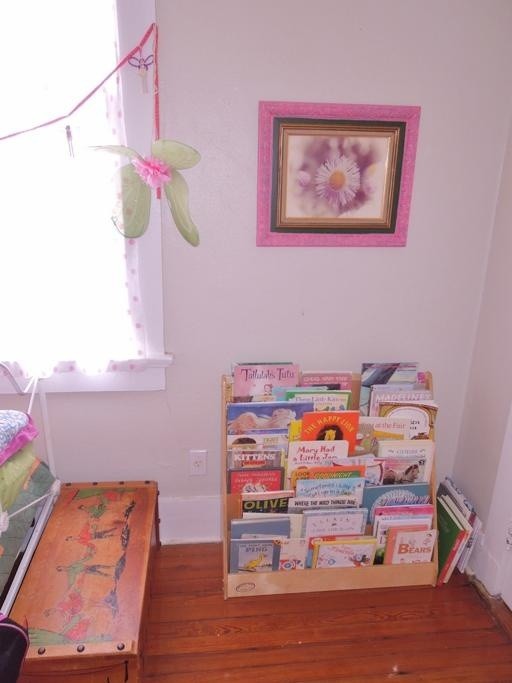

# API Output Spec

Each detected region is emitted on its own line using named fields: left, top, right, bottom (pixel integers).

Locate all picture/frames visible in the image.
left=255, top=100, right=422, bottom=253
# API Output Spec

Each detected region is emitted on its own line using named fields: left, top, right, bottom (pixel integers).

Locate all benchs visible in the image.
left=0, top=480, right=163, bottom=683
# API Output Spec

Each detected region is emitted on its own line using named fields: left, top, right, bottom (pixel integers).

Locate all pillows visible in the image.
left=1, top=409, right=40, bottom=466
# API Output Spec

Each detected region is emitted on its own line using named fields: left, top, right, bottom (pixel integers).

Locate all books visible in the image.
left=360, top=439, right=438, bottom=564
left=225, top=402, right=367, bottom=513
left=279, top=539, right=308, bottom=570
left=231, top=362, right=301, bottom=403
left=288, top=387, right=354, bottom=411
left=306, top=535, right=376, bottom=567
left=435, top=475, right=482, bottom=586
left=230, top=538, right=281, bottom=573
left=230, top=508, right=367, bottom=543
left=313, top=542, right=376, bottom=568
left=354, top=362, right=440, bottom=457
left=299, top=369, right=352, bottom=390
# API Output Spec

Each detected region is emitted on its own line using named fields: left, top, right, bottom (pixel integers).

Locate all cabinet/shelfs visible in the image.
left=223, top=371, right=440, bottom=599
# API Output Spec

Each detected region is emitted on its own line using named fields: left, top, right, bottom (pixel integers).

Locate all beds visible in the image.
left=0, top=357, right=59, bottom=622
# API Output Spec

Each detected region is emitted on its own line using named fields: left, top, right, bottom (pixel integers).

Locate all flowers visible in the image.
left=85, top=136, right=207, bottom=250
